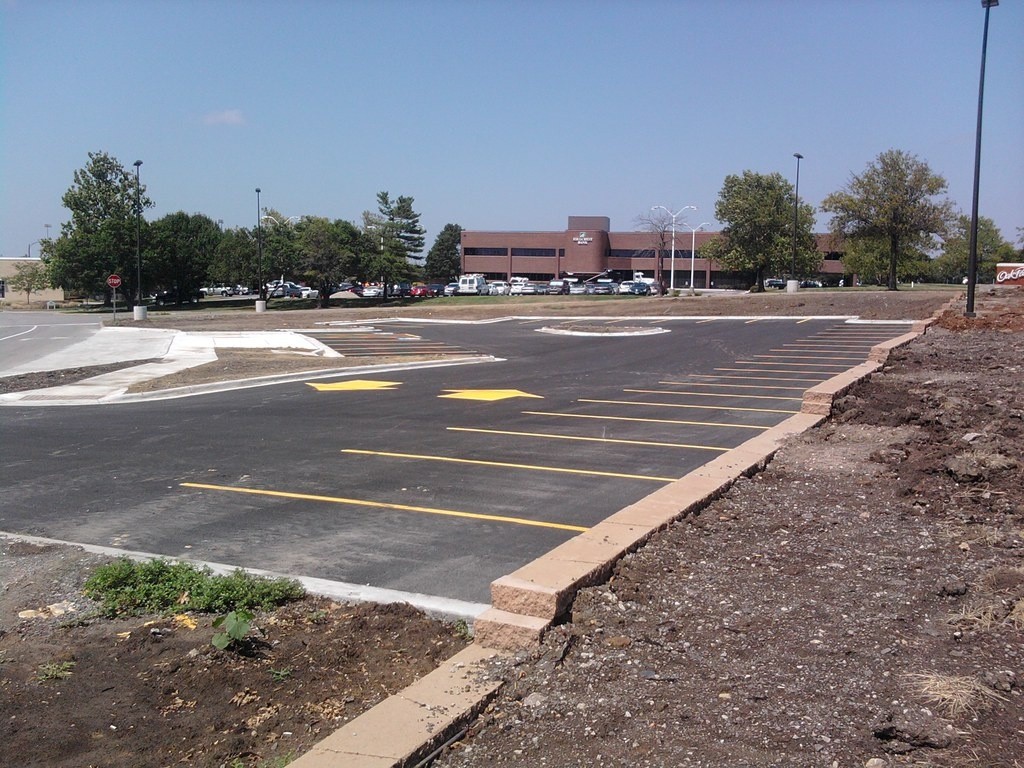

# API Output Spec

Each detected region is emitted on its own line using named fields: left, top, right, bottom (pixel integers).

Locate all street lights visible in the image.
left=133, top=159, right=147, bottom=321
left=680, top=221, right=712, bottom=288
left=260, top=214, right=299, bottom=278
left=367, top=225, right=384, bottom=282
left=961, top=1, right=1000, bottom=317
left=255, top=187, right=266, bottom=313
left=786, top=153, right=804, bottom=294
left=651, top=205, right=697, bottom=290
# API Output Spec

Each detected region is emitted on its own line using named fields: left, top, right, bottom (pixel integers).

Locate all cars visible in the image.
left=199, top=271, right=662, bottom=298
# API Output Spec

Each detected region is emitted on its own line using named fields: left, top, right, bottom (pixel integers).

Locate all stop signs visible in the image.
left=106, top=275, right=122, bottom=288
